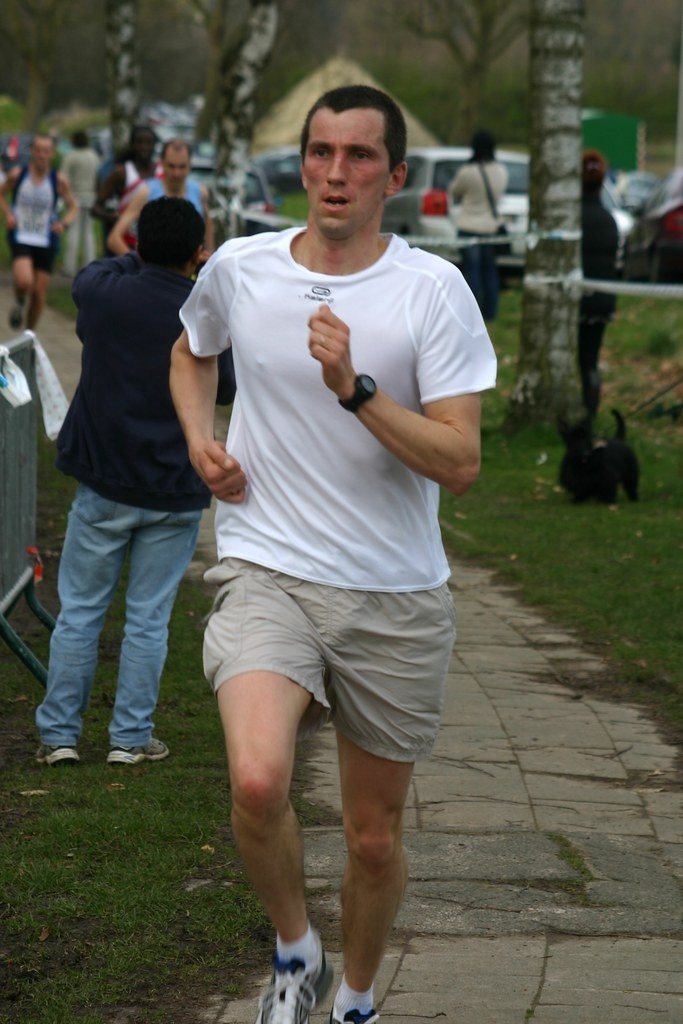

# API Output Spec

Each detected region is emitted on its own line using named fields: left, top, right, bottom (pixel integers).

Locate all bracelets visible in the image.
left=60, top=220, right=69, bottom=229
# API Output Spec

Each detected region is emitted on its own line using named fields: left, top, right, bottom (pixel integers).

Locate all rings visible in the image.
left=320, top=335, right=325, bottom=345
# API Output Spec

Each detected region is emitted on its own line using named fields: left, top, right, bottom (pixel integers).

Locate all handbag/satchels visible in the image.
left=494, top=224, right=512, bottom=257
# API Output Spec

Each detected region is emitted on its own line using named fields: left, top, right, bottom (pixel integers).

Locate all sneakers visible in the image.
left=107, top=736, right=169, bottom=765
left=36, top=744, right=79, bottom=765
left=329, top=1003, right=379, bottom=1024
left=255, top=930, right=333, bottom=1024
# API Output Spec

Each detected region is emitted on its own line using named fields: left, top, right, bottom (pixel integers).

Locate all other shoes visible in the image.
left=9, top=307, right=22, bottom=330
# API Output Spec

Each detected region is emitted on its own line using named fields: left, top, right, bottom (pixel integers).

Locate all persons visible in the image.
left=34, top=194, right=236, bottom=766
left=168, top=83, right=500, bottom=1024
left=108, top=139, right=214, bottom=266
left=86, top=125, right=159, bottom=257
left=577, top=149, right=619, bottom=418
left=61, top=129, right=103, bottom=276
left=0, top=134, right=80, bottom=331
left=447, top=125, right=510, bottom=324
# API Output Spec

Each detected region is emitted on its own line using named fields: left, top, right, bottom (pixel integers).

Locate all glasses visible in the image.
left=162, top=161, right=191, bottom=169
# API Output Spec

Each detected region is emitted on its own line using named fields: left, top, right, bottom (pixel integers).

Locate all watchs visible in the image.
left=338, top=374, right=377, bottom=414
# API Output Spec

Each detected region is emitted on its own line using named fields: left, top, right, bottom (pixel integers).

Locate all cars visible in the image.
left=138, top=105, right=683, bottom=290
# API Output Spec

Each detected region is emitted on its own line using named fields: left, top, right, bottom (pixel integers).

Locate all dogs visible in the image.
left=558, top=410, right=639, bottom=504
